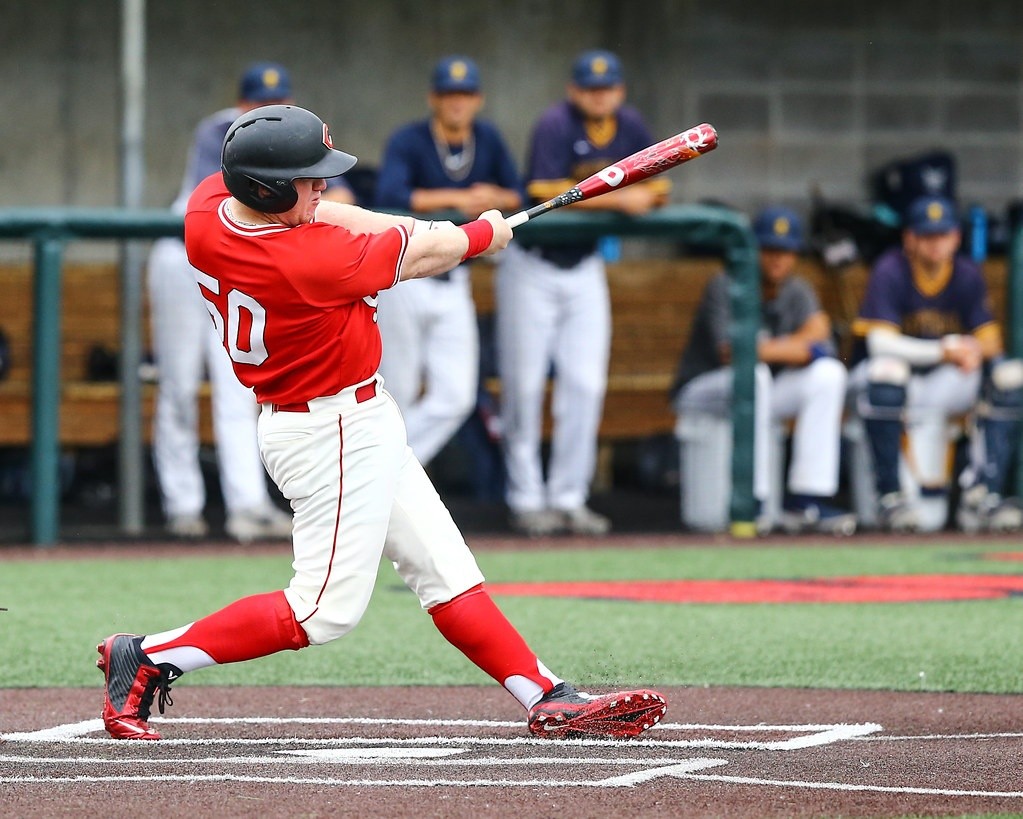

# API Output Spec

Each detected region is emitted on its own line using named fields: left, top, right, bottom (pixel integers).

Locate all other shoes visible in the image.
left=958, top=494, right=1021, bottom=533
left=563, top=507, right=611, bottom=535
left=509, top=509, right=563, bottom=533
left=225, top=504, right=295, bottom=543
left=781, top=497, right=858, bottom=533
left=169, top=513, right=209, bottom=539
left=889, top=494, right=948, bottom=533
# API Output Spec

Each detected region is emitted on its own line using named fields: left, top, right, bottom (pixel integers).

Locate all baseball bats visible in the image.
left=504, top=122, right=720, bottom=231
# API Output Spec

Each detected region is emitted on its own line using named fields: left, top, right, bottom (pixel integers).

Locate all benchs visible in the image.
left=0, top=242, right=1007, bottom=535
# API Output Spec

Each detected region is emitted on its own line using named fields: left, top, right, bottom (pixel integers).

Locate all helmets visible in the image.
left=221, top=103, right=357, bottom=215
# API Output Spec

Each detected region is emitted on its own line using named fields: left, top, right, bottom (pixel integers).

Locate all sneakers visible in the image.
left=96, top=633, right=184, bottom=740
left=529, top=682, right=668, bottom=740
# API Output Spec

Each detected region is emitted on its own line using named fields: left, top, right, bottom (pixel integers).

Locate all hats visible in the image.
left=909, top=198, right=958, bottom=235
left=753, top=207, right=802, bottom=250
left=238, top=63, right=292, bottom=101
left=432, top=55, right=480, bottom=92
left=571, top=49, right=623, bottom=88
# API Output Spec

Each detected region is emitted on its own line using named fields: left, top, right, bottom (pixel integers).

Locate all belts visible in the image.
left=271, top=380, right=375, bottom=413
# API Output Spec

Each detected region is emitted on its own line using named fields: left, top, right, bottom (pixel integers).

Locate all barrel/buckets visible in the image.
left=674, top=415, right=783, bottom=532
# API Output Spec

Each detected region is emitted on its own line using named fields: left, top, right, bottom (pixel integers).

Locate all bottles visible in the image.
left=968, top=207, right=989, bottom=263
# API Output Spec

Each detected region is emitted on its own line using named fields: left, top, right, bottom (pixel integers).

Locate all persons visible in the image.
left=147, top=60, right=353, bottom=536
left=96, top=106, right=665, bottom=739
left=495, top=49, right=668, bottom=531
left=668, top=207, right=855, bottom=537
left=375, top=55, right=524, bottom=465
left=850, top=199, right=1023, bottom=530
